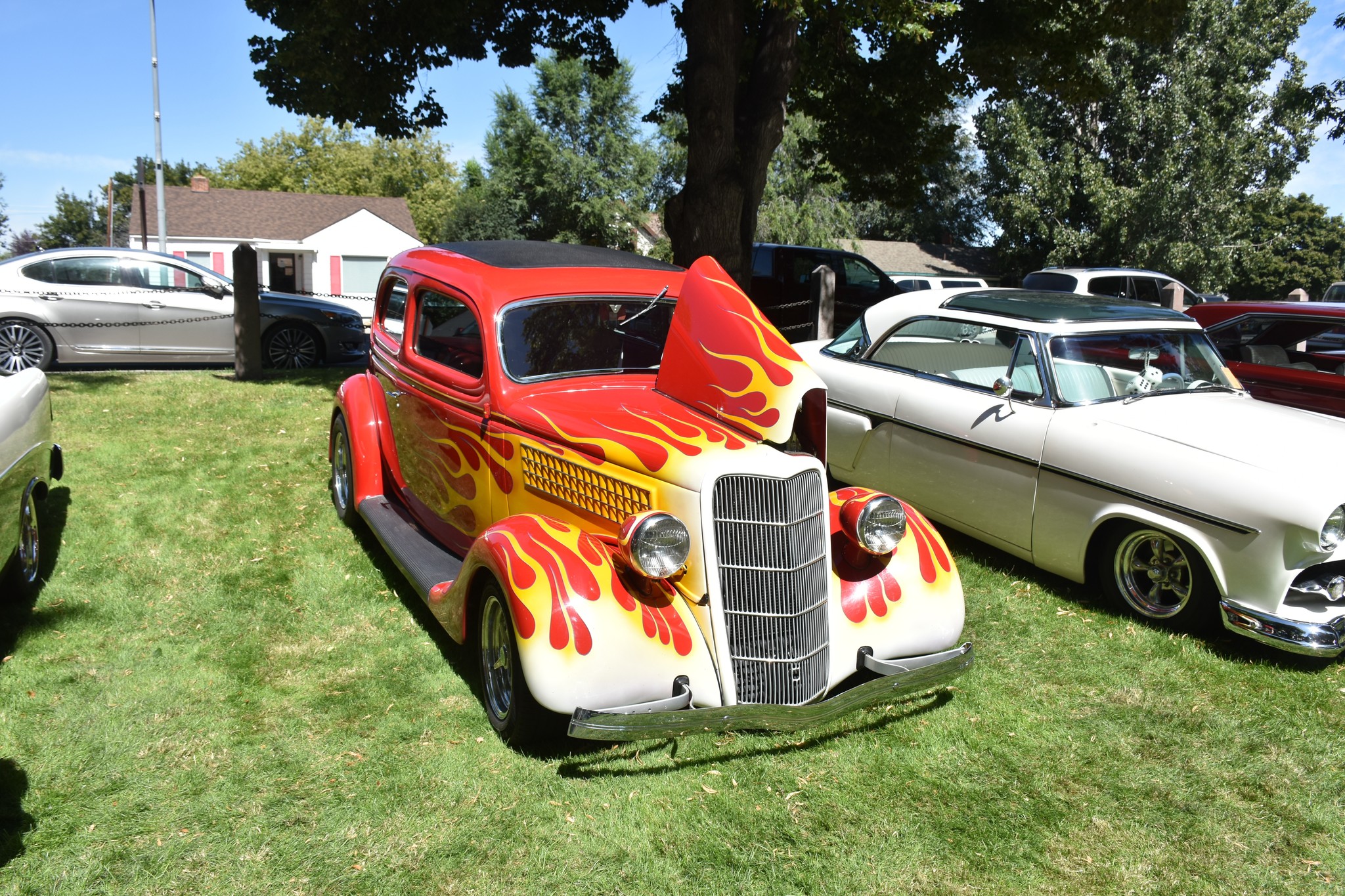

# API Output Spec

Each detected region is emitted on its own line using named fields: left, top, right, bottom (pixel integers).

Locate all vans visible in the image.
left=750, top=241, right=903, bottom=345
left=872, top=276, right=989, bottom=294
left=1022, top=265, right=1206, bottom=314
left=1322, top=282, right=1345, bottom=303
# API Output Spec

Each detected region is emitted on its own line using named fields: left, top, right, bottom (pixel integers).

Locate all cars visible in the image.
left=0, top=365, right=64, bottom=601
left=1028, top=300, right=1345, bottom=419
left=328, top=239, right=974, bottom=740
left=0, top=247, right=367, bottom=379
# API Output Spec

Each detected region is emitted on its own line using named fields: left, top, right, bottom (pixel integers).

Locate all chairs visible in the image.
left=1239, top=344, right=1290, bottom=365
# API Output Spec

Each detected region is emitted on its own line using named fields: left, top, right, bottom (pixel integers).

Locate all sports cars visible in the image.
left=781, top=287, right=1345, bottom=661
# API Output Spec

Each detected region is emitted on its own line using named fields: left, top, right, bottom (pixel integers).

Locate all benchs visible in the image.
left=937, top=365, right=1117, bottom=402
left=872, top=342, right=1012, bottom=373
